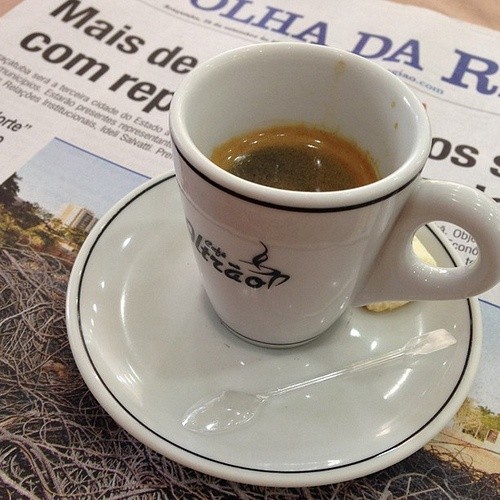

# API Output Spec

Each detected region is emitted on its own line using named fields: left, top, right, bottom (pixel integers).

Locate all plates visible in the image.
left=63, top=172, right=482, bottom=488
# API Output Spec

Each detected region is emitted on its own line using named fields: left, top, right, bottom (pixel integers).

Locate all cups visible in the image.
left=169, top=41, right=499, bottom=350
